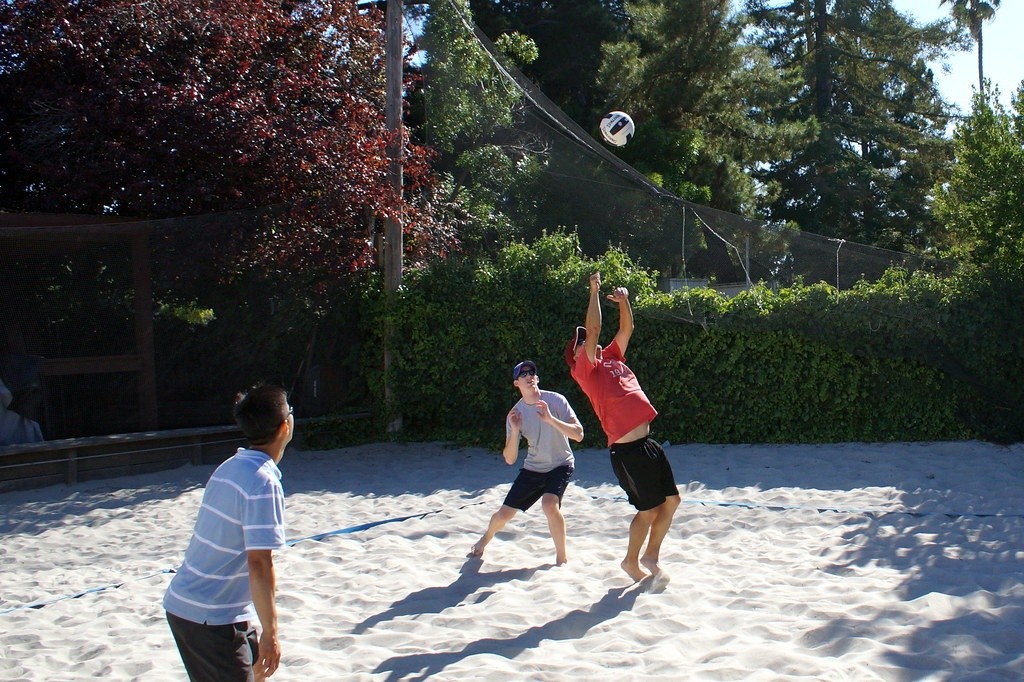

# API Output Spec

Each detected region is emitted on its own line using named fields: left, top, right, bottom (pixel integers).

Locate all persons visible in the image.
left=0, top=377, right=44, bottom=445
left=471, top=359, right=584, bottom=567
left=565, top=271, right=681, bottom=582
left=163, top=381, right=296, bottom=682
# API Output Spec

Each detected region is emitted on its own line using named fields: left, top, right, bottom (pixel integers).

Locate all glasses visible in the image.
left=576, top=338, right=586, bottom=345
left=518, top=369, right=537, bottom=377
left=277, top=405, right=294, bottom=433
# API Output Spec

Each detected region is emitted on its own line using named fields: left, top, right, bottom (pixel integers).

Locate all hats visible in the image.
left=565, top=326, right=587, bottom=367
left=513, top=360, right=537, bottom=380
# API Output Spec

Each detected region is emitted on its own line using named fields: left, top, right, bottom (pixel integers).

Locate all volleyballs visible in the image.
left=599, top=110, right=636, bottom=147
